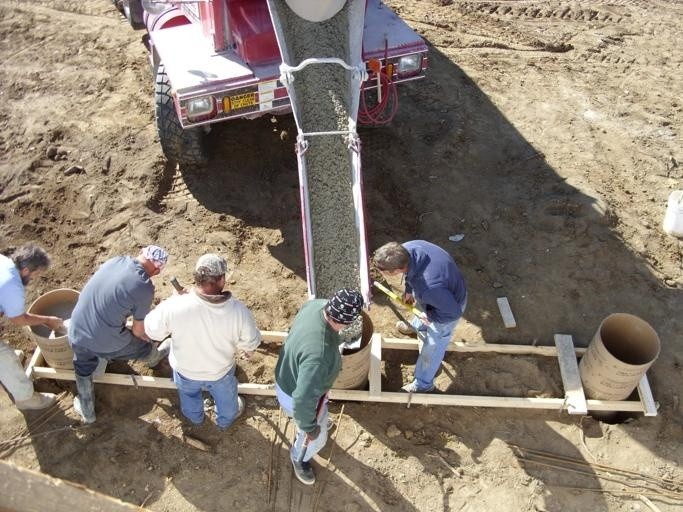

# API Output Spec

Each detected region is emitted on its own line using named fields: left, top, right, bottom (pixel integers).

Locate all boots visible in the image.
left=142, top=338, right=172, bottom=368
left=73, top=372, right=96, bottom=423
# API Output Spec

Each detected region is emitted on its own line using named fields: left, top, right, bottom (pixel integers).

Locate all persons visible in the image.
left=1, top=242, right=67, bottom=409
left=67, top=242, right=171, bottom=422
left=372, top=238, right=468, bottom=394
left=271, top=288, right=365, bottom=486
left=141, top=252, right=261, bottom=430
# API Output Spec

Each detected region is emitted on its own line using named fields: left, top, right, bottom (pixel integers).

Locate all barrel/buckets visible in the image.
left=334, top=311, right=375, bottom=390
left=579, top=312, right=662, bottom=420
left=662, top=189, right=682, bottom=240
left=28, top=288, right=81, bottom=370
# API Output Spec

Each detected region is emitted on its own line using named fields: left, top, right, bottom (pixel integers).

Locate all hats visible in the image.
left=196, top=253, right=228, bottom=277
left=145, top=245, right=168, bottom=269
left=325, top=287, right=364, bottom=325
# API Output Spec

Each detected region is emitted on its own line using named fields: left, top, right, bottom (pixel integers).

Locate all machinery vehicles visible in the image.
left=120, top=0, right=433, bottom=315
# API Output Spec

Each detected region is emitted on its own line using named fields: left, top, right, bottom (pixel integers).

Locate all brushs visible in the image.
left=368, top=58, right=382, bottom=103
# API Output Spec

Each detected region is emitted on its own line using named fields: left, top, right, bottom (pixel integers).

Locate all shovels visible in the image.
left=370, top=267, right=425, bottom=319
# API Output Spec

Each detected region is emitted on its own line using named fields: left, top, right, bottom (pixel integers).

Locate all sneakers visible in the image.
left=15, top=391, right=56, bottom=411
left=326, top=418, right=334, bottom=432
left=396, top=320, right=415, bottom=335
left=400, top=380, right=435, bottom=393
left=217, top=396, right=246, bottom=428
left=290, top=451, right=315, bottom=485
